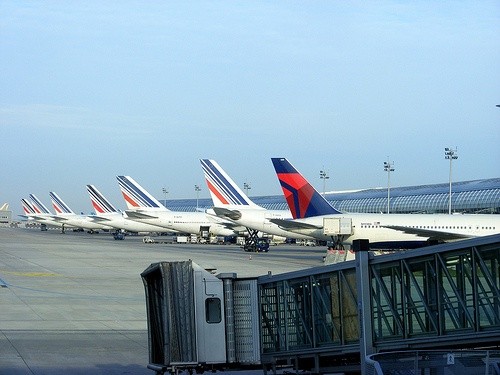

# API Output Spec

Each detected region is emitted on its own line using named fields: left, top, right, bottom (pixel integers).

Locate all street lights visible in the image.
left=319, top=170, right=329, bottom=197
left=445, top=147, right=459, bottom=214
left=384, top=161, right=396, bottom=214
left=161, top=186, right=169, bottom=206
left=194, top=184, right=201, bottom=212
left=244, top=182, right=252, bottom=197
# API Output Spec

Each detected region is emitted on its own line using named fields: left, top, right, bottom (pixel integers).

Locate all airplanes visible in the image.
left=197, top=157, right=500, bottom=264
left=18, top=174, right=326, bottom=247
